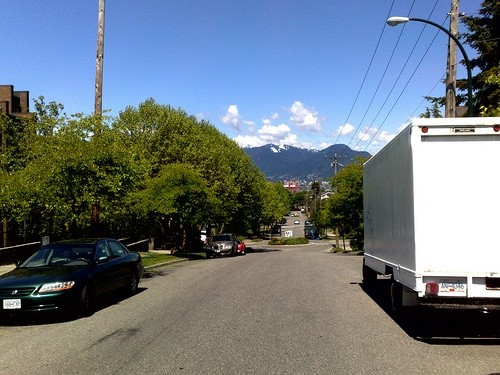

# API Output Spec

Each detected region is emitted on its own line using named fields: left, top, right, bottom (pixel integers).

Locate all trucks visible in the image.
left=361, top=116, right=500, bottom=324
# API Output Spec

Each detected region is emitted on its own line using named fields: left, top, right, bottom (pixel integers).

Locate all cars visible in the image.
left=204, top=233, right=247, bottom=259
left=0, top=237, right=145, bottom=323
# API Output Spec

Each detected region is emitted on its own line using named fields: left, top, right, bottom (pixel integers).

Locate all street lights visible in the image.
left=385, top=15, right=474, bottom=118
